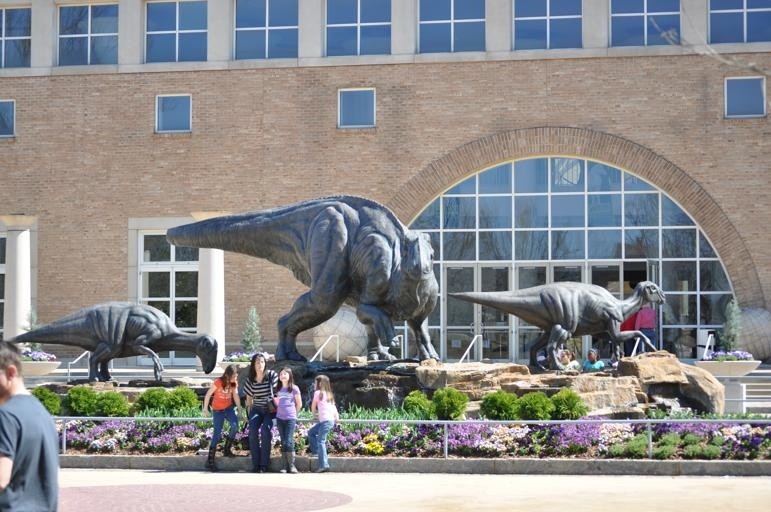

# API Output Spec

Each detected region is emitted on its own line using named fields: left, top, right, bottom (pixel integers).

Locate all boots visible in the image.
left=280, top=451, right=297, bottom=473
left=224, top=438, right=234, bottom=457
left=206, top=447, right=217, bottom=471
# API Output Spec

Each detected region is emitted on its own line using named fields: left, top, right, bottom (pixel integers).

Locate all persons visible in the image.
left=243, top=353, right=278, bottom=473
left=308, top=375, right=339, bottom=473
left=582, top=348, right=605, bottom=370
left=276, top=367, right=303, bottom=473
left=560, top=348, right=583, bottom=372
left=620, top=311, right=639, bottom=357
left=203, top=365, right=242, bottom=472
left=0, top=341, right=59, bottom=512
left=635, top=303, right=656, bottom=352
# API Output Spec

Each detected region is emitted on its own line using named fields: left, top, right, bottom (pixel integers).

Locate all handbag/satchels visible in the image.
left=268, top=400, right=276, bottom=413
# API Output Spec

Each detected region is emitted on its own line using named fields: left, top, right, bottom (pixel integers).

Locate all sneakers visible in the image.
left=316, top=465, right=329, bottom=473
